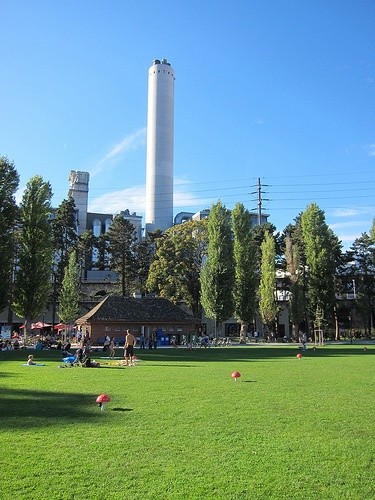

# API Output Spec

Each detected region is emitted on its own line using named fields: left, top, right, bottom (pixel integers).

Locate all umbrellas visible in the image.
left=19, top=322, right=52, bottom=335
left=54, top=322, right=69, bottom=329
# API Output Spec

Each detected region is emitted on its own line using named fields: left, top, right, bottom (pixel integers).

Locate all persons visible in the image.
left=63, top=330, right=111, bottom=367
left=149, top=336, right=152, bottom=350
left=139, top=333, right=145, bottom=349
left=12, top=331, right=19, bottom=338
left=153, top=335, right=157, bottom=349
left=27, top=355, right=35, bottom=365
left=0, top=337, right=63, bottom=352
left=253, top=330, right=259, bottom=342
left=109, top=337, right=115, bottom=359
left=124, top=329, right=137, bottom=366
